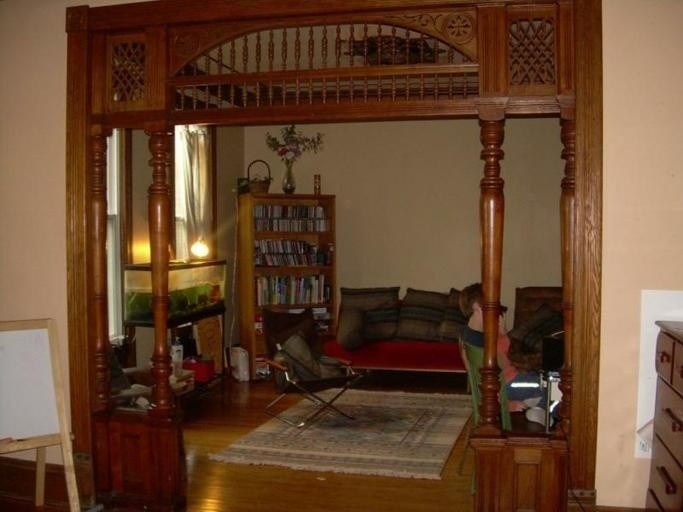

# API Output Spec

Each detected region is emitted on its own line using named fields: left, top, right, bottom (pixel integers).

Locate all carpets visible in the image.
left=207, top=385, right=475, bottom=481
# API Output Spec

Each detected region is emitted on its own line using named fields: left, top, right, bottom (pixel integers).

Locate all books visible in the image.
left=252, top=203, right=333, bottom=376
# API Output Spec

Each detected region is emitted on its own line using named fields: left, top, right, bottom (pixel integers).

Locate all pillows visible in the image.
left=502, top=302, right=566, bottom=369
left=336, top=287, right=475, bottom=351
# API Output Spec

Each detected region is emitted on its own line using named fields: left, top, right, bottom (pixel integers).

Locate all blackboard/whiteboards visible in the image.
left=0, top=318, right=70, bottom=454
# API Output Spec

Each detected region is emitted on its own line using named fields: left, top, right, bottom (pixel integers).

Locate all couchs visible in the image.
left=319, top=287, right=508, bottom=391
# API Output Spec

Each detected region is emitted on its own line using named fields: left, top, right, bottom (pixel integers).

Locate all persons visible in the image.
left=458, top=281, right=564, bottom=429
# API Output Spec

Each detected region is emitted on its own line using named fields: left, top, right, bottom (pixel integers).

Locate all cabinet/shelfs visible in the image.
left=122, top=255, right=229, bottom=422
left=644, top=318, right=683, bottom=511
left=238, top=192, right=338, bottom=382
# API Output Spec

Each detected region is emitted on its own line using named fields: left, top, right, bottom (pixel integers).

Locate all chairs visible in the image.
left=260, top=351, right=364, bottom=428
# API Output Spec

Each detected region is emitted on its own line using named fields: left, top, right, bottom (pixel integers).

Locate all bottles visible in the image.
left=170, top=336, right=183, bottom=376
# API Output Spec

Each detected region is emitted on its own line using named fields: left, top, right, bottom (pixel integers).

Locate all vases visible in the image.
left=282, top=166, right=296, bottom=194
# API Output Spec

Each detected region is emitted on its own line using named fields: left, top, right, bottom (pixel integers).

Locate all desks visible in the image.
left=468, top=408, right=568, bottom=512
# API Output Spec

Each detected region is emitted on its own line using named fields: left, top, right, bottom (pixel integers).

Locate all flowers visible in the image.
left=262, top=123, right=325, bottom=183
